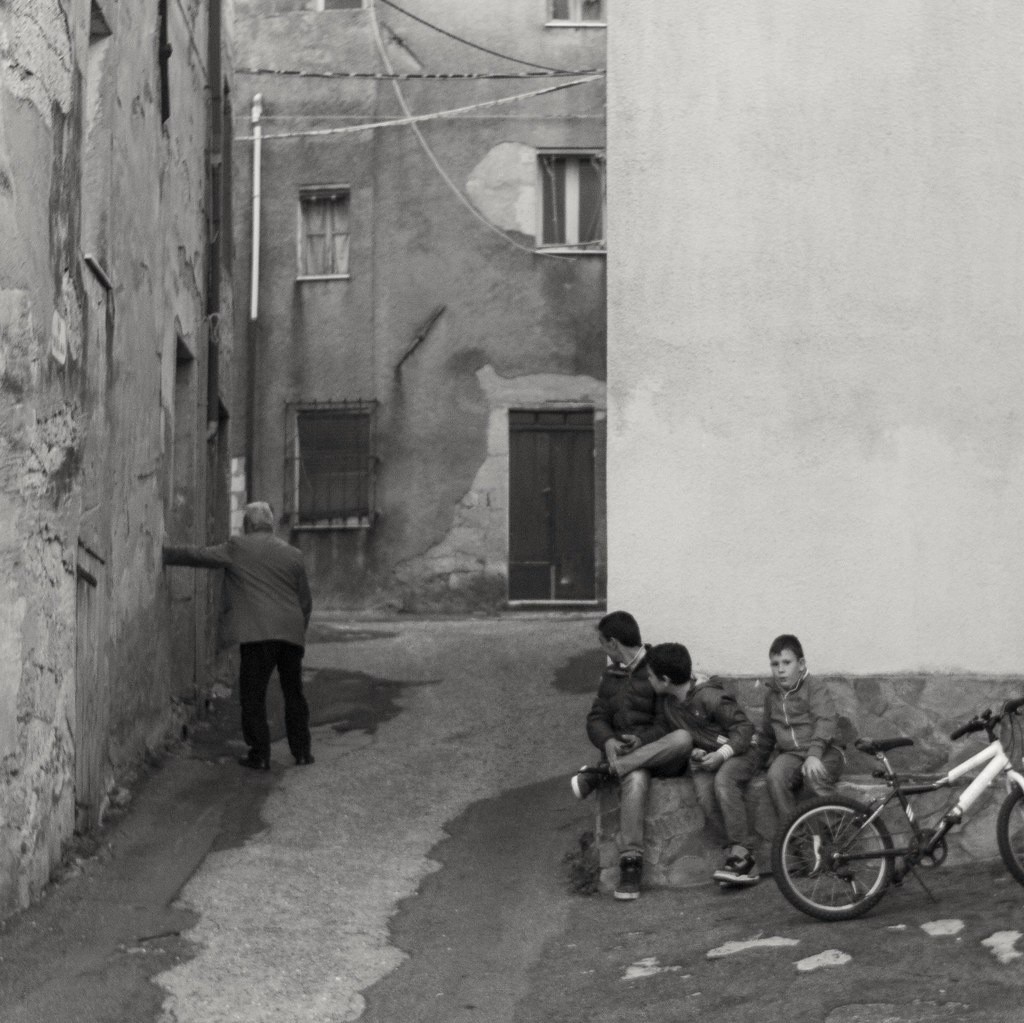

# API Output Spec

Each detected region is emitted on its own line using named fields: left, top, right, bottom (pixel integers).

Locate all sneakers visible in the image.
left=614, top=856, right=642, bottom=900
left=571, top=764, right=615, bottom=800
left=797, top=833, right=821, bottom=877
left=713, top=843, right=761, bottom=889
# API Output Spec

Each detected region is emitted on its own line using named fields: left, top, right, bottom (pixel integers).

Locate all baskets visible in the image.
left=1000, top=714, right=1024, bottom=772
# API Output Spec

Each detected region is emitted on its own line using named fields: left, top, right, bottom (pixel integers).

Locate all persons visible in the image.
left=646, top=642, right=773, bottom=889
left=570, top=611, right=692, bottom=900
left=750, top=634, right=848, bottom=878
left=161, top=501, right=315, bottom=770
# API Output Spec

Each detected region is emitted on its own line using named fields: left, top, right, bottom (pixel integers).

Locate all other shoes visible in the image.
left=296, top=752, right=315, bottom=765
left=238, top=754, right=270, bottom=769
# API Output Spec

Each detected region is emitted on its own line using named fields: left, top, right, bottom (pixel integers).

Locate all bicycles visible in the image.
left=771, top=695, right=1024, bottom=925
